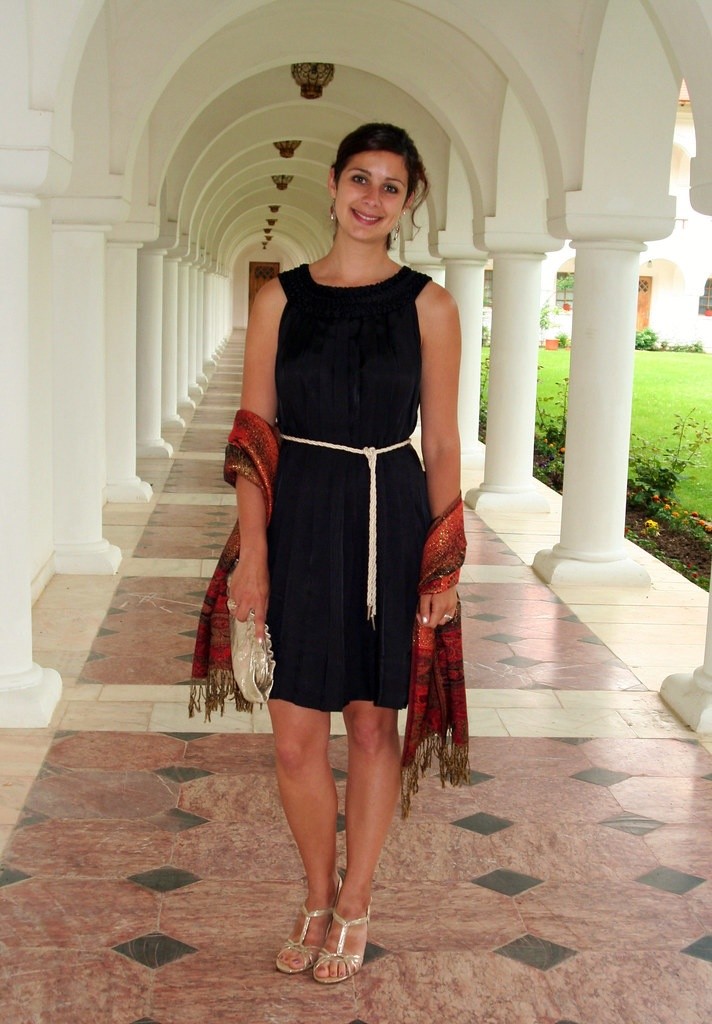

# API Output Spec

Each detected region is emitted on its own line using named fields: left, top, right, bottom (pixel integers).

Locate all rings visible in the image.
left=444, top=614, right=453, bottom=621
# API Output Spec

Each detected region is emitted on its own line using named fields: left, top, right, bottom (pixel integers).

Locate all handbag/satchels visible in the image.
left=227, top=599, right=276, bottom=706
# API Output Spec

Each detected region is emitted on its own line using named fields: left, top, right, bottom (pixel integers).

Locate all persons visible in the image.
left=225, top=125, right=467, bottom=984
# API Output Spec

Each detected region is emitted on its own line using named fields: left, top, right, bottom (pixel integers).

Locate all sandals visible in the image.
left=275, top=877, right=373, bottom=983
